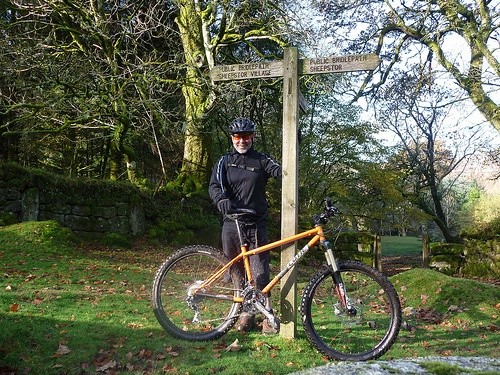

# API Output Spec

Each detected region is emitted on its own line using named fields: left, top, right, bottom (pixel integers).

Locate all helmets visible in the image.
left=228, top=118, right=255, bottom=132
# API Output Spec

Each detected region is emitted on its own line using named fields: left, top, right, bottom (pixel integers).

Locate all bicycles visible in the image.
left=151, top=197, right=402, bottom=361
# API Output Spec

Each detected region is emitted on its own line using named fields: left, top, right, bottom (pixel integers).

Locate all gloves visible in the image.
left=218, top=198, right=236, bottom=215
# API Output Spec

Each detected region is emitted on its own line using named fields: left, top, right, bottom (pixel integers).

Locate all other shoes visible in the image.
left=236, top=314, right=256, bottom=332
left=262, top=319, right=278, bottom=335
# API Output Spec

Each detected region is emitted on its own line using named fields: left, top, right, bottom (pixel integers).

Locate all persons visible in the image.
left=208, top=117, right=282, bottom=334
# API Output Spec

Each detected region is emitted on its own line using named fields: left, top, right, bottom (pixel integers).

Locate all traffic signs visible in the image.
left=211, top=53, right=384, bottom=81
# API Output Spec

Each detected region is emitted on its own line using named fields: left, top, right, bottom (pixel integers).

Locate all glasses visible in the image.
left=233, top=134, right=253, bottom=140
